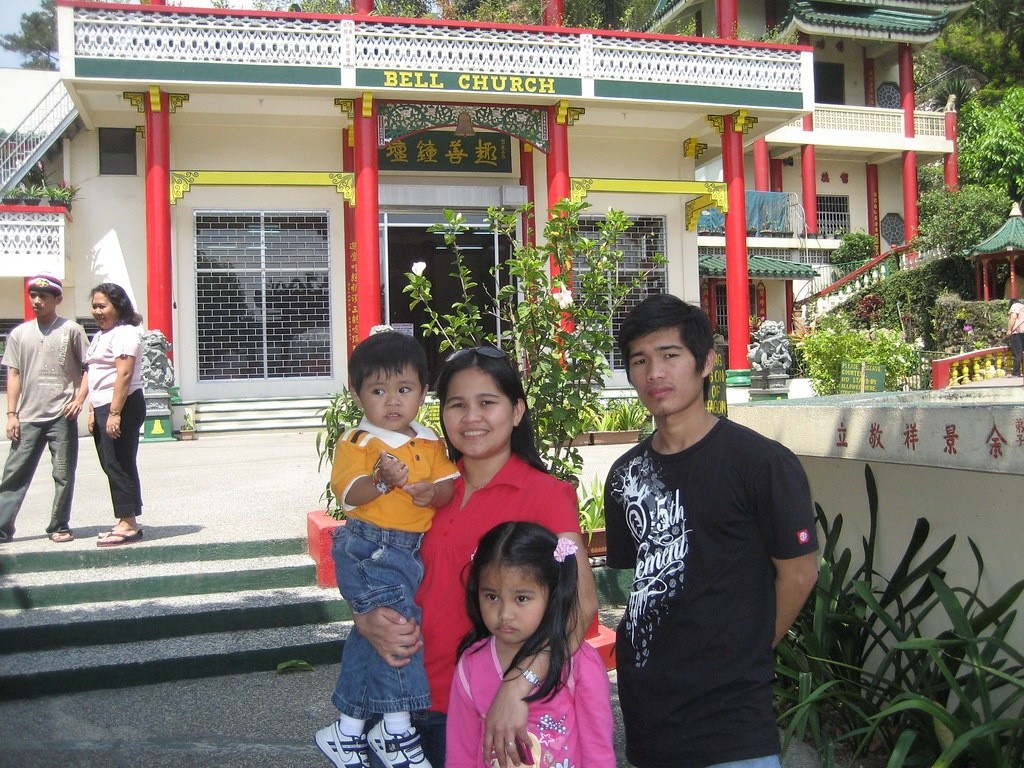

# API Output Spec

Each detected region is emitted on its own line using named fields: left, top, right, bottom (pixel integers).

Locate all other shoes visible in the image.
left=52, top=532, right=73, bottom=542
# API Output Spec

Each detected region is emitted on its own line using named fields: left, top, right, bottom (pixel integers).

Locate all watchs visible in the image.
left=373, top=468, right=393, bottom=496
left=516, top=665, right=541, bottom=687
left=108, top=411, right=120, bottom=416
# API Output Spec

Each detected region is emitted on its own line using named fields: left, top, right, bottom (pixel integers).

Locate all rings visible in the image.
left=112, top=429, right=116, bottom=431
left=506, top=742, right=515, bottom=745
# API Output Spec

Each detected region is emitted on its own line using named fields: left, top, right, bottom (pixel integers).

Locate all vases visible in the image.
left=49, top=196, right=72, bottom=213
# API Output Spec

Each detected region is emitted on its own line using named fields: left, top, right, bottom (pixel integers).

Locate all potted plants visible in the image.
left=0, top=185, right=24, bottom=204
left=578, top=475, right=607, bottom=557
left=179, top=414, right=197, bottom=440
left=759, top=211, right=794, bottom=238
left=548, top=402, right=649, bottom=449
left=22, top=184, right=46, bottom=206
left=697, top=224, right=758, bottom=237
left=800, top=226, right=845, bottom=239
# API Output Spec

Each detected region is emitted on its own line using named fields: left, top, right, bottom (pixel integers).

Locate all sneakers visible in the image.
left=314, top=720, right=371, bottom=768
left=367, top=719, right=433, bottom=768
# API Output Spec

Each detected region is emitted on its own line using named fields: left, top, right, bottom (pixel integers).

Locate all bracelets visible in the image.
left=90, top=412, right=94, bottom=413
left=7, top=412, right=15, bottom=415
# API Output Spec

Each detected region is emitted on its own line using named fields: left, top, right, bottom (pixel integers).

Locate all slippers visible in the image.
left=98, top=529, right=144, bottom=547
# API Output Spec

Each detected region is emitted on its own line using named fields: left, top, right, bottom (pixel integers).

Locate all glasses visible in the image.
left=445, top=345, right=519, bottom=379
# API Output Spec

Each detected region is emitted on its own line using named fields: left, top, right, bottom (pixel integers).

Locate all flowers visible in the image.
left=44, top=180, right=86, bottom=204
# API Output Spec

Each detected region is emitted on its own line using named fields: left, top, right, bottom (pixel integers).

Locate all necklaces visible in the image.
left=37, top=315, right=58, bottom=342
left=87, top=333, right=104, bottom=356
left=466, top=479, right=486, bottom=489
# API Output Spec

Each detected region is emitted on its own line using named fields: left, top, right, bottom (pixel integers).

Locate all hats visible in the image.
left=28, top=275, right=63, bottom=296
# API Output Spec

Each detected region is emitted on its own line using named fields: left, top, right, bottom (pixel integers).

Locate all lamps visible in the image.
left=785, top=156, right=794, bottom=166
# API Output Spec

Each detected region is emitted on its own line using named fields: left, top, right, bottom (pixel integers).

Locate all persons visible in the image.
left=313, top=331, right=461, bottom=768
left=1006, top=299, right=1024, bottom=377
left=86, top=283, right=146, bottom=545
left=604, top=294, right=820, bottom=768
left=0, top=270, right=91, bottom=542
left=349, top=347, right=601, bottom=768
left=443, top=521, right=618, bottom=768
